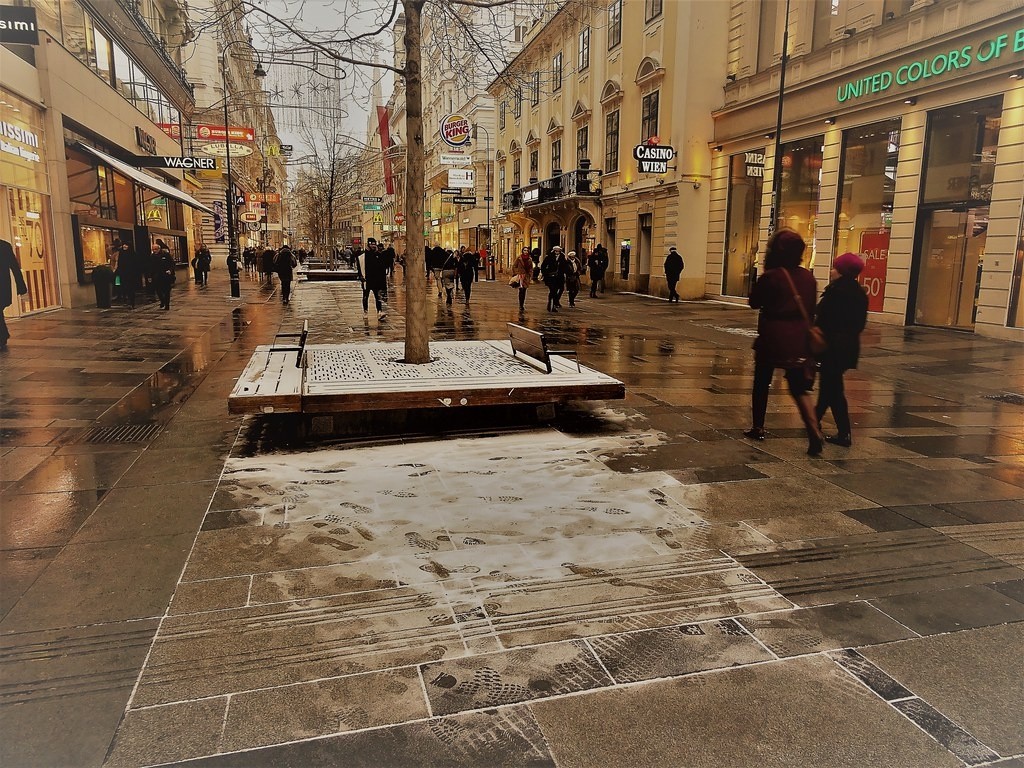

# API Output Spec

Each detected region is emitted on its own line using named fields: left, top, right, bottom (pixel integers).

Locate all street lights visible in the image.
left=280, top=179, right=297, bottom=247
left=223, top=40, right=268, bottom=298
left=464, top=125, right=490, bottom=254
left=262, top=134, right=287, bottom=248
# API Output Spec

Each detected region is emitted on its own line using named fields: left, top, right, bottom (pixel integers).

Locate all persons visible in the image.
left=740, top=226, right=868, bottom=455
left=157, top=239, right=170, bottom=252
left=195, top=243, right=212, bottom=290
left=274, top=245, right=297, bottom=300
left=400, top=249, right=407, bottom=276
left=426, top=241, right=487, bottom=306
left=357, top=238, right=396, bottom=319
left=110, top=241, right=136, bottom=309
left=588, top=244, right=609, bottom=298
left=664, top=247, right=684, bottom=302
left=147, top=244, right=175, bottom=309
left=512, top=247, right=533, bottom=310
left=292, top=248, right=296, bottom=255
left=243, top=243, right=276, bottom=280
left=532, top=246, right=583, bottom=312
left=0, top=238, right=27, bottom=350
left=299, top=248, right=314, bottom=264
left=333, top=246, right=362, bottom=266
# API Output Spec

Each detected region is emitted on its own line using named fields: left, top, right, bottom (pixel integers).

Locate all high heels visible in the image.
left=742, top=426, right=766, bottom=440
left=805, top=437, right=827, bottom=456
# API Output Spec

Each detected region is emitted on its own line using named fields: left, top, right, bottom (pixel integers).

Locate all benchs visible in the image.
left=508, top=321, right=593, bottom=377
left=243, top=318, right=309, bottom=371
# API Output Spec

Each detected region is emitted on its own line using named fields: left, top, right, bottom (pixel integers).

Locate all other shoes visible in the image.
left=546, top=290, right=601, bottom=312
left=669, top=297, right=672, bottom=303
left=675, top=294, right=680, bottom=302
left=160, top=302, right=165, bottom=309
left=164, top=306, right=169, bottom=310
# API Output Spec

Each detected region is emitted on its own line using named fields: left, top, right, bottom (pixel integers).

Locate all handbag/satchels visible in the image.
left=797, top=358, right=819, bottom=392
left=509, top=273, right=524, bottom=289
left=806, top=324, right=830, bottom=357
left=236, top=261, right=243, bottom=272
left=111, top=273, right=121, bottom=286
left=191, top=258, right=199, bottom=269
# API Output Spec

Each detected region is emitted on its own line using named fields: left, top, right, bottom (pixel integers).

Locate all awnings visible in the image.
left=74, top=140, right=219, bottom=217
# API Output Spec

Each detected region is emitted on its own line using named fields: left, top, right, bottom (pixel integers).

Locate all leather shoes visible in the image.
left=826, top=431, right=851, bottom=447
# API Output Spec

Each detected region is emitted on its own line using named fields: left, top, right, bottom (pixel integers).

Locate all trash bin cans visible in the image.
left=92, top=265, right=114, bottom=307
left=485, top=256, right=495, bottom=280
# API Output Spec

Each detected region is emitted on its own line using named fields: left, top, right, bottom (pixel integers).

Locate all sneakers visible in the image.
left=376, top=310, right=387, bottom=321
left=362, top=312, right=368, bottom=319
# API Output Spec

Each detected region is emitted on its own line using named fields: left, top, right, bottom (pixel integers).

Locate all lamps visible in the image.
left=764, top=134, right=773, bottom=139
left=844, top=27, right=857, bottom=36
left=693, top=179, right=699, bottom=189
left=781, top=54, right=790, bottom=61
left=656, top=177, right=664, bottom=185
left=713, top=146, right=722, bottom=152
left=904, top=98, right=916, bottom=105
left=886, top=12, right=895, bottom=20
left=825, top=118, right=835, bottom=124
left=147, top=206, right=163, bottom=222
left=621, top=183, right=628, bottom=190
left=725, top=74, right=736, bottom=82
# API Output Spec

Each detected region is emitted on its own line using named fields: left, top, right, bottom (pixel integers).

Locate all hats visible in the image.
left=367, top=238, right=376, bottom=245
left=550, top=245, right=563, bottom=253
left=834, top=253, right=865, bottom=279
